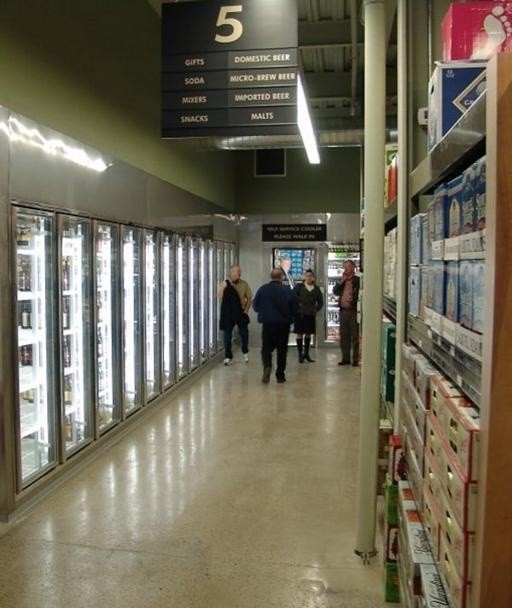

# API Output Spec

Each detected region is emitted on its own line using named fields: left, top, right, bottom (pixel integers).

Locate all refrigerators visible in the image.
left=63, top=212, right=93, bottom=464
left=324, top=241, right=361, bottom=342
left=98, top=218, right=122, bottom=436
left=124, top=223, right=239, bottom=419
left=17, top=209, right=57, bottom=494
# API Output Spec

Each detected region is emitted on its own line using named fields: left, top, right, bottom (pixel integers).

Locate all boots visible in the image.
left=304, top=336, right=315, bottom=362
left=297, top=338, right=304, bottom=363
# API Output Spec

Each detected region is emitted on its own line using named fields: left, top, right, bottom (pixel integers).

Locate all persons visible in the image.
left=332, top=260, right=359, bottom=367
left=280, top=257, right=293, bottom=286
left=217, top=264, right=253, bottom=366
left=291, top=269, right=323, bottom=363
left=252, top=268, right=296, bottom=383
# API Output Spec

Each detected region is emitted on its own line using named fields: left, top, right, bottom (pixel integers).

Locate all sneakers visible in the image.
left=277, top=378, right=286, bottom=383
left=338, top=361, right=350, bottom=365
left=240, top=351, right=249, bottom=363
left=224, top=357, right=233, bottom=365
left=262, top=367, right=272, bottom=383
left=352, top=361, right=358, bottom=366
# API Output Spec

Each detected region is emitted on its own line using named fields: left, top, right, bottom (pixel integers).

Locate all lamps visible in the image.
left=296, top=67, right=320, bottom=164
